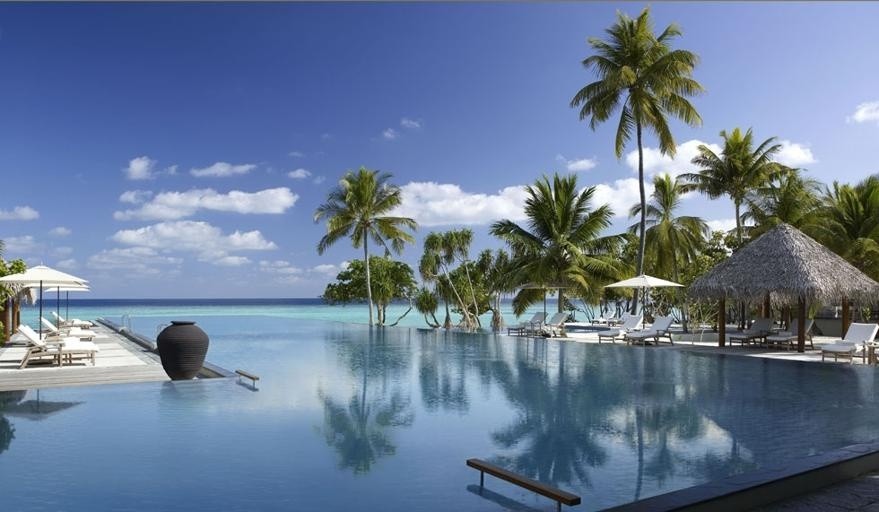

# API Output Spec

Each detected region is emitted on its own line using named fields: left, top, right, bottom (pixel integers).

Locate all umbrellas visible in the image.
left=601, top=274, right=686, bottom=330
left=513, top=279, right=580, bottom=324
left=17, top=283, right=90, bottom=331
left=1, top=267, right=91, bottom=343
left=43, top=288, right=90, bottom=324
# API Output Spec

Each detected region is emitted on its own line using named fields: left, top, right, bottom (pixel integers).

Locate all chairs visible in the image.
left=507, top=312, right=573, bottom=342
left=821, top=322, right=879, bottom=366
left=14, top=310, right=101, bottom=368
left=591, top=310, right=675, bottom=348
left=728, top=316, right=815, bottom=352
